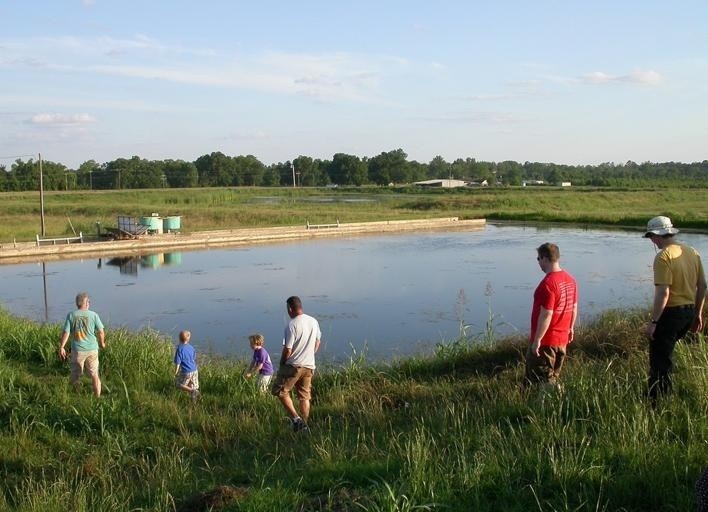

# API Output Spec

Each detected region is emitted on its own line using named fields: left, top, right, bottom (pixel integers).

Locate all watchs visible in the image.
left=651, top=316, right=659, bottom=325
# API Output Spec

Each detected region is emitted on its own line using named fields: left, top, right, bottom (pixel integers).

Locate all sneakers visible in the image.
left=291, top=420, right=307, bottom=432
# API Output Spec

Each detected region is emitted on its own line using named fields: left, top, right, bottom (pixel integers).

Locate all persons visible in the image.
left=272, top=296, right=322, bottom=433
left=642, top=216, right=708, bottom=413
left=245, top=334, right=274, bottom=397
left=524, top=242, right=578, bottom=388
left=59, top=291, right=105, bottom=399
left=173, top=329, right=200, bottom=407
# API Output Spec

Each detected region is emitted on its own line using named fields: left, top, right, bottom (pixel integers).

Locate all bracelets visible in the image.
left=59, top=346, right=65, bottom=349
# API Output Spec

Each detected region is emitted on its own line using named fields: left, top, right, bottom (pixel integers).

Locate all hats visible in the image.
left=641, top=215, right=679, bottom=239
